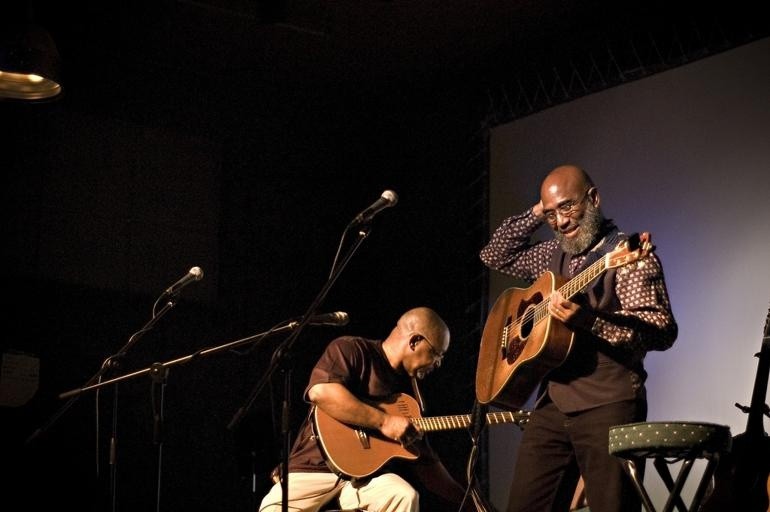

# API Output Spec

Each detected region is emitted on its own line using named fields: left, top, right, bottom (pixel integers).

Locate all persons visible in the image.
left=476, top=163, right=681, bottom=510
left=256, top=306, right=454, bottom=510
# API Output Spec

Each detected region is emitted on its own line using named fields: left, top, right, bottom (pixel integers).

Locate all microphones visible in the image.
left=350, top=190, right=398, bottom=225
left=165, top=266, right=204, bottom=295
left=300, top=311, right=350, bottom=328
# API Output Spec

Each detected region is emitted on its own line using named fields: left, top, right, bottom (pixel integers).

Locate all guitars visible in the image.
left=698, top=311, right=770, bottom=512
left=476, top=231, right=656, bottom=408
left=310, top=392, right=533, bottom=485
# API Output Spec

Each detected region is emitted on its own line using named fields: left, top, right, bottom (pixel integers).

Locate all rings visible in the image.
left=555, top=307, right=560, bottom=315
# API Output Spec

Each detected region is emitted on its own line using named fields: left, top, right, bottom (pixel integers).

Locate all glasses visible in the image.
left=544, top=199, right=577, bottom=224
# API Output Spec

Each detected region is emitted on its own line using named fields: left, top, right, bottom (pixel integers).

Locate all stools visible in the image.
left=608, top=421, right=732, bottom=512
